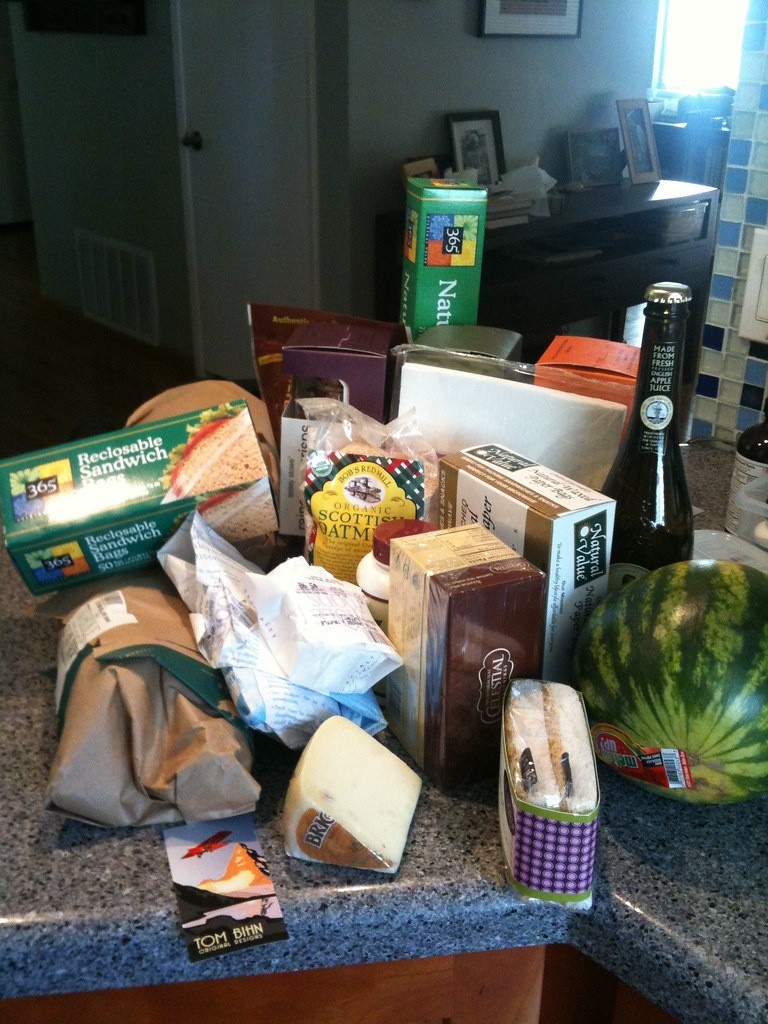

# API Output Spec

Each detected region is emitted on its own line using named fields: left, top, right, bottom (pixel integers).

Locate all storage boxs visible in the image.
left=385, top=522, right=549, bottom=792
left=279, top=320, right=395, bottom=536
left=397, top=179, right=488, bottom=345
left=0, top=400, right=281, bottom=594
left=413, top=324, right=523, bottom=368
left=535, top=334, right=641, bottom=446
left=439, top=442, right=618, bottom=686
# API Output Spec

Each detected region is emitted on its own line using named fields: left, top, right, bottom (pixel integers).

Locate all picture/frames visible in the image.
left=480, top=0, right=585, bottom=39
left=443, top=108, right=505, bottom=185
left=398, top=155, right=441, bottom=189
left=563, top=127, right=624, bottom=190
left=616, top=97, right=662, bottom=186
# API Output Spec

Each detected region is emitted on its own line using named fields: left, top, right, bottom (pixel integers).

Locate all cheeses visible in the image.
left=282, top=714, right=422, bottom=873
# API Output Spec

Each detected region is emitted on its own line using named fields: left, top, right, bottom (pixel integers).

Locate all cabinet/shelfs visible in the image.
left=373, top=180, right=719, bottom=383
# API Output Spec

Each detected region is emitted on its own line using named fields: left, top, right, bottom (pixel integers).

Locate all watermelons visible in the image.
left=571, top=557, right=768, bottom=805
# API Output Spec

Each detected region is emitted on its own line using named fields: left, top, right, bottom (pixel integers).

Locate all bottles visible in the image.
left=356, top=518, right=441, bottom=711
left=600, top=281, right=694, bottom=594
left=724, top=393, right=768, bottom=551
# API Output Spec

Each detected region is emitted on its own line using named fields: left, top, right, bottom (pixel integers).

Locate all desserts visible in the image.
left=156, top=400, right=280, bottom=543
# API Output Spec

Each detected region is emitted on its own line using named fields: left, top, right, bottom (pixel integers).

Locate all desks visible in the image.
left=652, top=117, right=732, bottom=200
